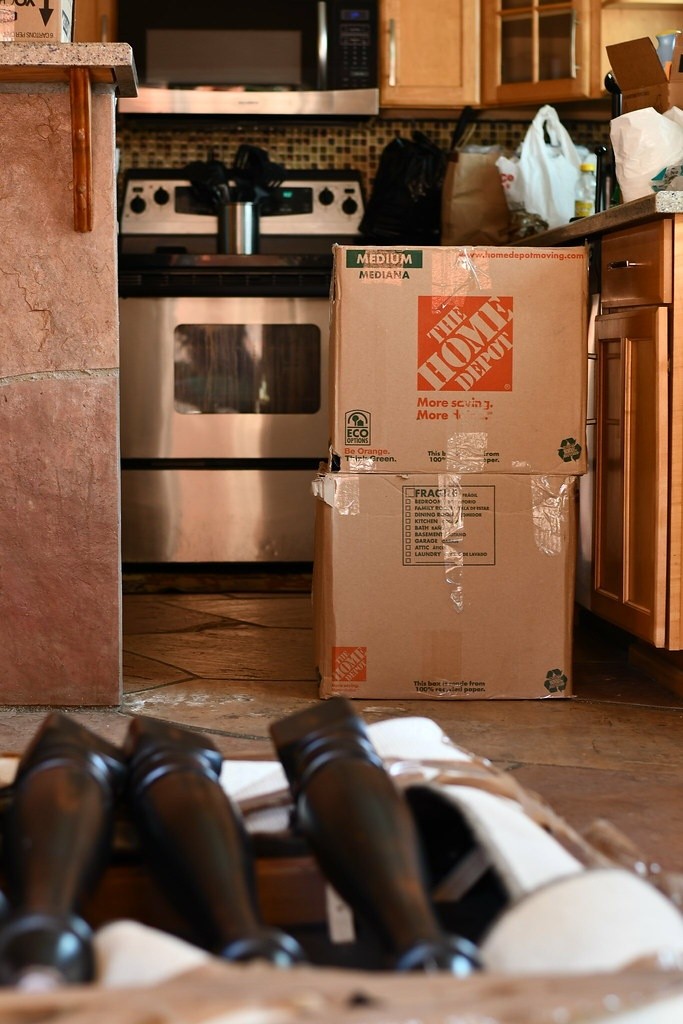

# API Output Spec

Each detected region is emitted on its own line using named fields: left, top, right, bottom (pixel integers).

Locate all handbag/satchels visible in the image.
left=493, top=106, right=595, bottom=233
left=440, top=117, right=512, bottom=247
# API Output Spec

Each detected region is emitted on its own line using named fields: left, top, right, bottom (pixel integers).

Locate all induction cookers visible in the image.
left=118, top=166, right=368, bottom=295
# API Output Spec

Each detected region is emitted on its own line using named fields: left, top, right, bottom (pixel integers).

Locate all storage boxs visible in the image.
left=314, top=473, right=576, bottom=703
left=325, top=234, right=590, bottom=476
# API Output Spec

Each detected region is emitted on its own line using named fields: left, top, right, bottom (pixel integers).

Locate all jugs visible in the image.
left=655, top=28, right=681, bottom=79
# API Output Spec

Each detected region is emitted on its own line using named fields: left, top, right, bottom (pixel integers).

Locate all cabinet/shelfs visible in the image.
left=584, top=213, right=683, bottom=653
left=379, top=0, right=683, bottom=118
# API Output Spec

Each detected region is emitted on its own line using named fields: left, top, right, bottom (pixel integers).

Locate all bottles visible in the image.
left=573, top=163, right=596, bottom=218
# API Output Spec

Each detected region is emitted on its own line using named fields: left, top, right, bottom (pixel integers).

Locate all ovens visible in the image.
left=120, top=299, right=333, bottom=587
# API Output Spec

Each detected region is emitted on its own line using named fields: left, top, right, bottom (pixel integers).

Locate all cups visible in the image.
left=222, top=202, right=259, bottom=256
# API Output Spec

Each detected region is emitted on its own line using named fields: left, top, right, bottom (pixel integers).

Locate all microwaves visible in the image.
left=117, top=1, right=380, bottom=90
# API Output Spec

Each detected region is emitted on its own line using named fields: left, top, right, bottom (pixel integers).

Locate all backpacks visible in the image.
left=361, top=131, right=444, bottom=245
